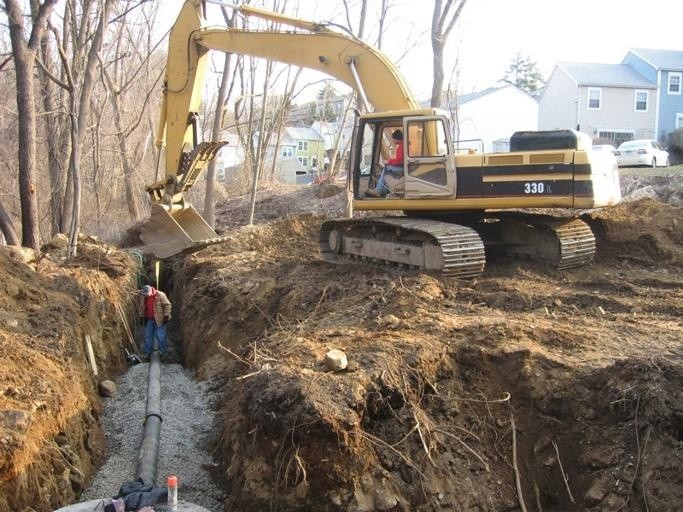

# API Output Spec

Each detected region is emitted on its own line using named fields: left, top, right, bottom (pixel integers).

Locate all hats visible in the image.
left=392, top=129, right=403, bottom=139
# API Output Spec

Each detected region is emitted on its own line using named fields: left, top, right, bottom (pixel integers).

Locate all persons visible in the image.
left=139, top=285, right=173, bottom=362
left=368, top=129, right=404, bottom=197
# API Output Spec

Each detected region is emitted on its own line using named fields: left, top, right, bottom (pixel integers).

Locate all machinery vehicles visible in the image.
left=138, top=0, right=621, bottom=282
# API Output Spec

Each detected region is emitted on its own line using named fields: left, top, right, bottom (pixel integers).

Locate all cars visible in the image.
left=616, top=140, right=671, bottom=169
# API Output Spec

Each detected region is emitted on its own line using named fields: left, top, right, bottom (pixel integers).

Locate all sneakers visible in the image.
left=368, top=188, right=382, bottom=197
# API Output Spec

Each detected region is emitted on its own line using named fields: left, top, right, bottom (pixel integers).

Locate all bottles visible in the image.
left=167, top=475, right=178, bottom=506
